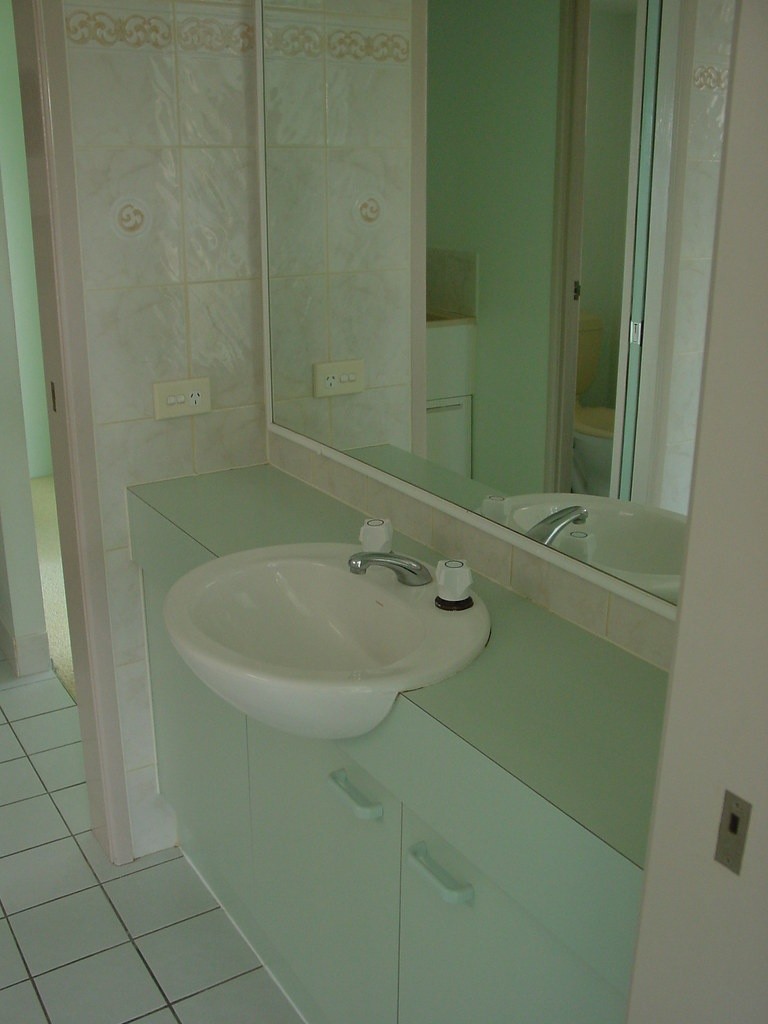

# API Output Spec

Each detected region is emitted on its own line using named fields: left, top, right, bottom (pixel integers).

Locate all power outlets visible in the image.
left=152, top=379, right=212, bottom=422
left=311, top=358, right=364, bottom=399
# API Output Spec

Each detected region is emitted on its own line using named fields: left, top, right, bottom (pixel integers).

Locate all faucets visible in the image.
left=348, top=552, right=433, bottom=587
left=525, top=505, right=588, bottom=547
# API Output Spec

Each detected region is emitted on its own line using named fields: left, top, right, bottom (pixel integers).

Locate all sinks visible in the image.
left=474, top=492, right=687, bottom=607
left=163, top=543, right=492, bottom=740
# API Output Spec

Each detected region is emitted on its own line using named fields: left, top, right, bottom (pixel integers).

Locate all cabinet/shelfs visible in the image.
left=127, top=468, right=626, bottom=1024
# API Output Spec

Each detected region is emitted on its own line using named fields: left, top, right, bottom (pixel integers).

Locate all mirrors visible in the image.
left=246, top=0, right=743, bottom=666
